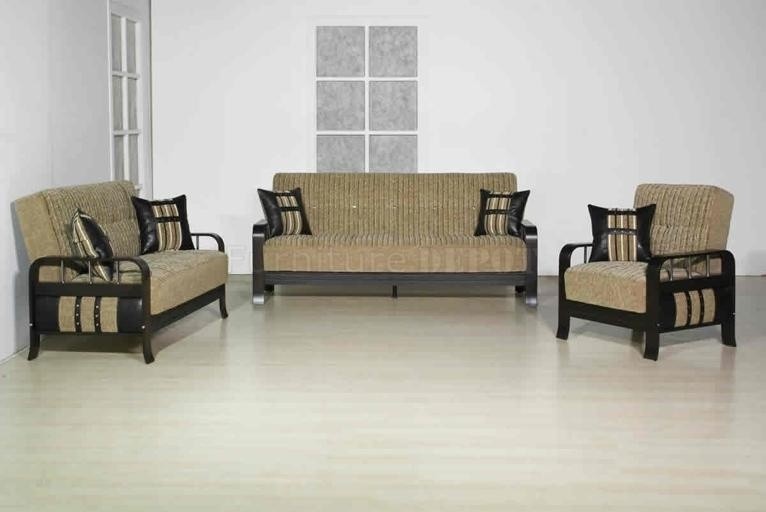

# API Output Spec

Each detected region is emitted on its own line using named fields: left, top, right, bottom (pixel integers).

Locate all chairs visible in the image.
left=559, top=183, right=739, bottom=359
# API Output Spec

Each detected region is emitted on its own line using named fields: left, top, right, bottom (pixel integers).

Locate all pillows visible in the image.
left=473, top=187, right=530, bottom=238
left=71, top=207, right=112, bottom=282
left=129, top=190, right=195, bottom=256
left=584, top=200, right=657, bottom=264
left=256, top=186, right=312, bottom=238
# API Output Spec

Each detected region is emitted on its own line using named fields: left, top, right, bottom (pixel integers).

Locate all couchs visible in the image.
left=250, top=170, right=540, bottom=312
left=15, top=177, right=229, bottom=365
